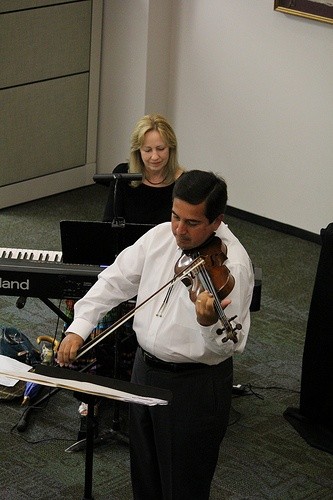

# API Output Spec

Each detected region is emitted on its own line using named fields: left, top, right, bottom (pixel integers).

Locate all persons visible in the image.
left=55, top=170, right=255, bottom=500
left=102, top=113, right=185, bottom=225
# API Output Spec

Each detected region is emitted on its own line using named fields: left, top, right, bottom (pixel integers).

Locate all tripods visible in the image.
left=66, top=327, right=129, bottom=453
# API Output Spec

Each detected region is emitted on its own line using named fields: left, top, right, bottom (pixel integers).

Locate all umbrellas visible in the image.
left=21, top=336, right=60, bottom=405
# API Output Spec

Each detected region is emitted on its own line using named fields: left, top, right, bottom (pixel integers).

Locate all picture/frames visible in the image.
left=273, top=0, right=333, bottom=24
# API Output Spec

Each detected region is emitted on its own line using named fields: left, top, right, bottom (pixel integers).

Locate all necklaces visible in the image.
left=144, top=176, right=167, bottom=185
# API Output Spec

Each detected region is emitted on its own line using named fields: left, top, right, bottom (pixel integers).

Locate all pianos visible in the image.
left=0, top=247, right=262, bottom=438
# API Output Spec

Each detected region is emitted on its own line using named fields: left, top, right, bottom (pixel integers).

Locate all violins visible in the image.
left=175, top=236, right=243, bottom=344
